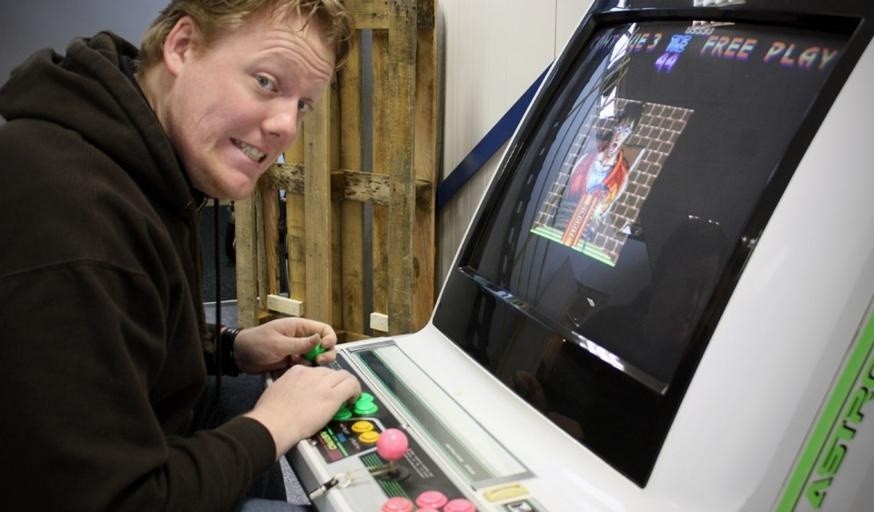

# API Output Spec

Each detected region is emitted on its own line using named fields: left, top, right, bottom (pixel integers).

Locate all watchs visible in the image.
left=218, top=326, right=245, bottom=379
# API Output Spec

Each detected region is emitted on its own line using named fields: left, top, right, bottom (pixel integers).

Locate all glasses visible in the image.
left=613, top=126, right=632, bottom=135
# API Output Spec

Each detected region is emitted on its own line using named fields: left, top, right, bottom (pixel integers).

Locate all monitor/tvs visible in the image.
left=455, top=7, right=868, bottom=397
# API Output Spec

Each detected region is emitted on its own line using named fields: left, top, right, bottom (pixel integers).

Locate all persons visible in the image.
left=551, top=101, right=647, bottom=244
left=0, top=0, right=363, bottom=512
